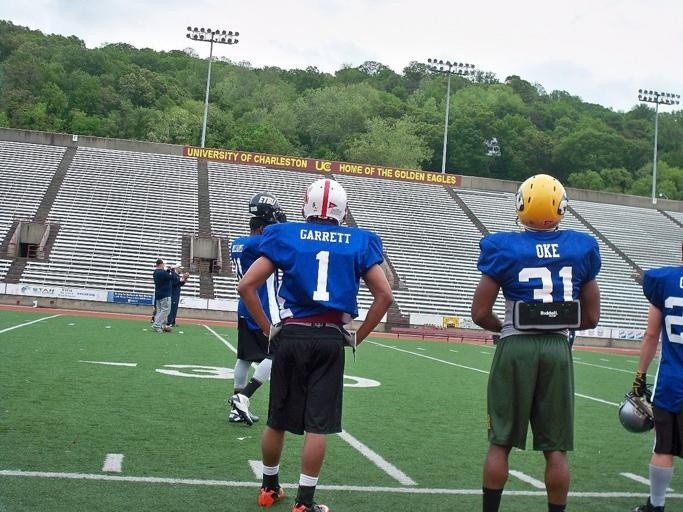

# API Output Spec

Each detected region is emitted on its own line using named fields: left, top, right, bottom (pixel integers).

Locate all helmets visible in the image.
left=517, top=174, right=568, bottom=230
left=618, top=390, right=654, bottom=432
left=303, top=178, right=347, bottom=224
left=249, top=193, right=281, bottom=221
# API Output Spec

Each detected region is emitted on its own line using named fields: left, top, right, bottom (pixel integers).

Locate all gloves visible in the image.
left=632, top=372, right=647, bottom=397
left=262, top=323, right=282, bottom=354
left=272, top=211, right=286, bottom=224
left=343, top=327, right=358, bottom=348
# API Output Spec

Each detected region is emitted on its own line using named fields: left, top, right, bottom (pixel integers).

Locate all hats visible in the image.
left=175, top=264, right=184, bottom=270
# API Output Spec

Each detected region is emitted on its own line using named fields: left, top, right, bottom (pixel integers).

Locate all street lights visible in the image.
left=633, top=86, right=679, bottom=199
left=424, top=56, right=478, bottom=175
left=183, top=24, right=241, bottom=149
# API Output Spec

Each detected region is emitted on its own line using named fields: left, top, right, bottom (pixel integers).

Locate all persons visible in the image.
left=149, top=297, right=157, bottom=322
left=234, top=177, right=394, bottom=512
left=226, top=191, right=286, bottom=425
left=166, top=263, right=188, bottom=327
left=152, top=260, right=174, bottom=332
left=618, top=243, right=682, bottom=512
left=469, top=174, right=601, bottom=512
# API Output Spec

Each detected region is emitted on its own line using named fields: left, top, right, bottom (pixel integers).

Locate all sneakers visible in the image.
left=258, top=486, right=284, bottom=507
left=632, top=497, right=665, bottom=512
left=292, top=503, right=330, bottom=512
left=227, top=392, right=259, bottom=426
